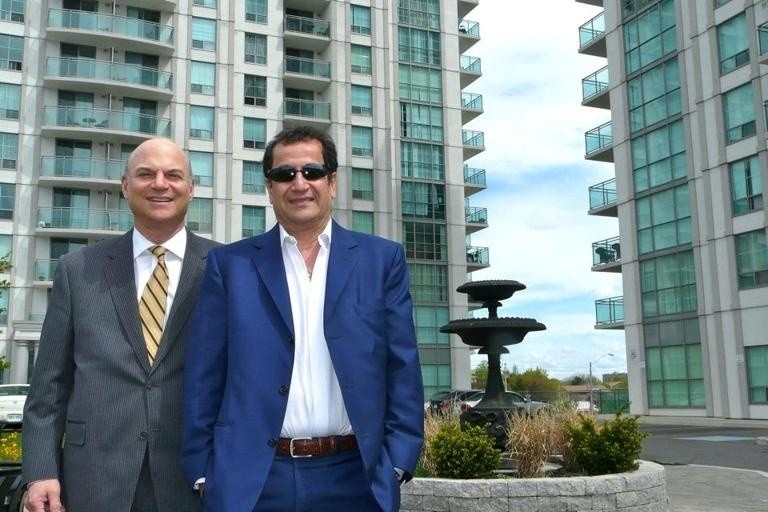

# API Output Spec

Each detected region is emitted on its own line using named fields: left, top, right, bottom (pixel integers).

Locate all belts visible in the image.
left=278, top=435, right=355, bottom=458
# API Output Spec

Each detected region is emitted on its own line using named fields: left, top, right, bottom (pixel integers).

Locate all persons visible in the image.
left=18, top=135, right=226, bottom=512
left=179, top=126, right=426, bottom=512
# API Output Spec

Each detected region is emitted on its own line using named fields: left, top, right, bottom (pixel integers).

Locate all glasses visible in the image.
left=265, top=163, right=328, bottom=182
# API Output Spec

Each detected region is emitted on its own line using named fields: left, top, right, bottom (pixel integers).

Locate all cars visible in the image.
left=422, top=388, right=556, bottom=423
left=572, top=400, right=600, bottom=416
left=0, top=383, right=31, bottom=430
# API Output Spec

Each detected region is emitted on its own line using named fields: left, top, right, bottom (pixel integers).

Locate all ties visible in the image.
left=138, top=245, right=169, bottom=367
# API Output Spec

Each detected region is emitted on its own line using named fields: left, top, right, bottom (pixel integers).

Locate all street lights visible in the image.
left=589, top=353, right=614, bottom=415
